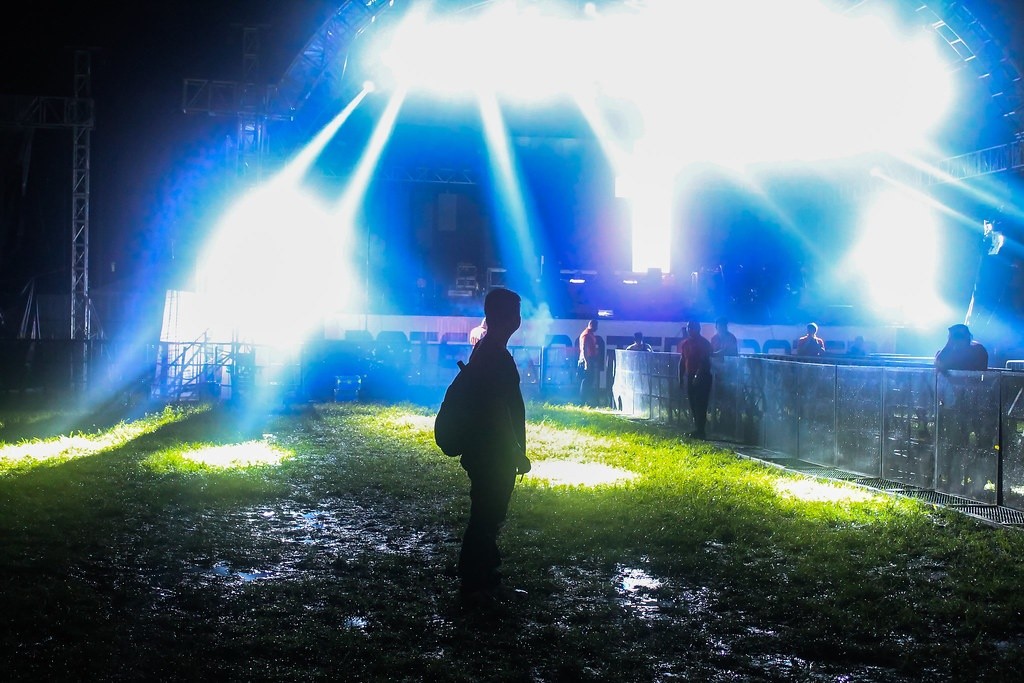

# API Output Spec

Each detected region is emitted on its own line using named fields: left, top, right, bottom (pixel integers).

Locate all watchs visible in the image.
left=506, top=440, right=522, bottom=451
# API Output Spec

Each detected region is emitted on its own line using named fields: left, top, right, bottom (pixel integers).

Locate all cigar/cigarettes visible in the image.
left=519, top=473, right=524, bottom=482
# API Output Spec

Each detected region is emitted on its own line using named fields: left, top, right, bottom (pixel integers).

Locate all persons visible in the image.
left=626, top=331, right=655, bottom=353
left=710, top=317, right=739, bottom=358
left=459, top=287, right=531, bottom=611
left=796, top=322, right=827, bottom=355
left=934, top=324, right=988, bottom=371
left=846, top=336, right=866, bottom=355
left=677, top=319, right=712, bottom=438
left=575, top=319, right=600, bottom=408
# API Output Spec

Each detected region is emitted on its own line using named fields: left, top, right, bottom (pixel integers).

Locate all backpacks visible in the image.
left=435, top=360, right=480, bottom=457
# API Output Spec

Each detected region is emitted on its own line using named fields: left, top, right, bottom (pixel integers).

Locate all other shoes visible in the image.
left=491, top=585, right=528, bottom=602
left=459, top=592, right=507, bottom=614
